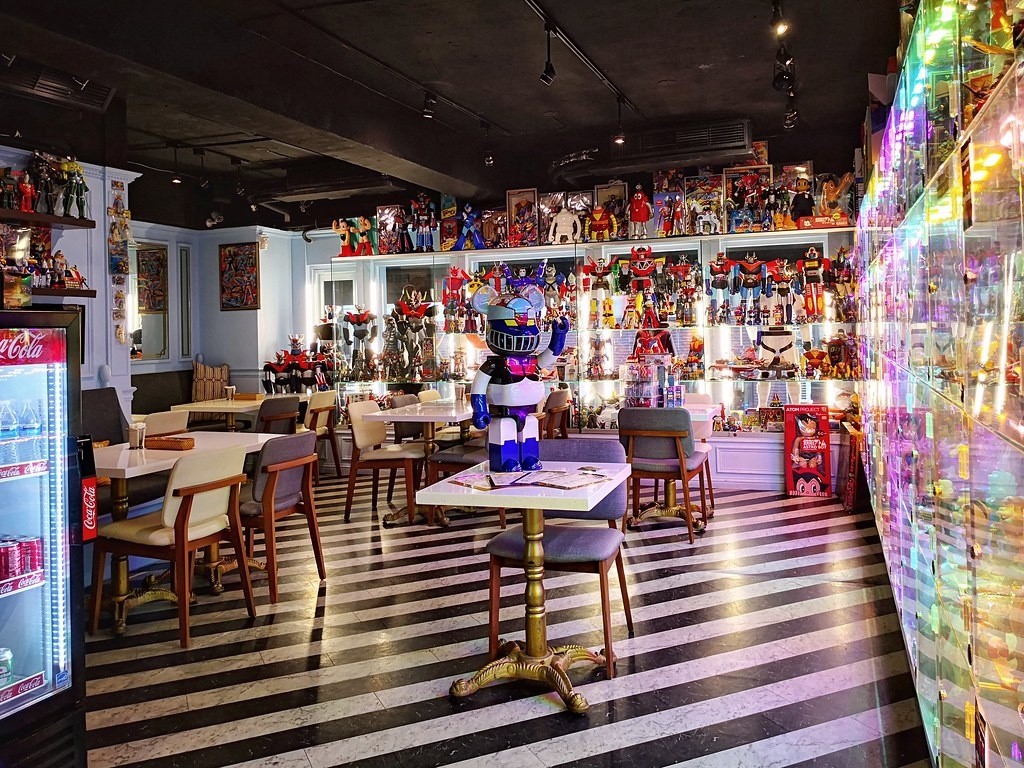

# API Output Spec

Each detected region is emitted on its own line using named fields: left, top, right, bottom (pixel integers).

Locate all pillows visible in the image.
left=188, top=359, right=232, bottom=420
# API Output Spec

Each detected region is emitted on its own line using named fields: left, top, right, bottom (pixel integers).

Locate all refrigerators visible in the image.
left=0, top=309, right=98, bottom=768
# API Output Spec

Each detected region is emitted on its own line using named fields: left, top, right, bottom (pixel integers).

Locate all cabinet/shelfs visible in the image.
left=327, top=227, right=856, bottom=431
left=0, top=208, right=98, bottom=299
left=854, top=0, right=1024, bottom=768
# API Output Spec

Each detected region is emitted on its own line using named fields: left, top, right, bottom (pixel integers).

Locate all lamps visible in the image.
left=422, top=87, right=512, bottom=167
left=524, top=0, right=649, bottom=145
left=162, top=135, right=245, bottom=195
left=768, top=0, right=800, bottom=129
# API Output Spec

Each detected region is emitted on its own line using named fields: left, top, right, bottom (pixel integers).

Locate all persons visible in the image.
left=792, top=414, right=824, bottom=471
left=332, top=217, right=354, bottom=257
left=350, top=214, right=373, bottom=256
left=820, top=173, right=852, bottom=215
left=790, top=177, right=816, bottom=220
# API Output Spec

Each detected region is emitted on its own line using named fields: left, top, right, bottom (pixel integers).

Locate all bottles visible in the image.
left=19, top=399, right=42, bottom=462
left=0, top=400, right=19, bottom=465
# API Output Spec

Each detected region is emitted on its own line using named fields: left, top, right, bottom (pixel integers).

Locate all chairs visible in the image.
left=485, top=438, right=634, bottom=680
left=297, top=388, right=343, bottom=484
left=465, top=398, right=546, bottom=445
left=386, top=393, right=463, bottom=503
left=343, top=399, right=440, bottom=523
left=617, top=407, right=708, bottom=544
left=427, top=446, right=505, bottom=529
left=542, top=388, right=572, bottom=439
left=87, top=443, right=256, bottom=649
left=254, top=398, right=299, bottom=434
left=416, top=389, right=487, bottom=492
left=188, top=430, right=328, bottom=605
left=141, top=409, right=190, bottom=438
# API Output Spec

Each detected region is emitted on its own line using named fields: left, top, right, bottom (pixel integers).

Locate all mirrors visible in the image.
left=129, top=236, right=173, bottom=364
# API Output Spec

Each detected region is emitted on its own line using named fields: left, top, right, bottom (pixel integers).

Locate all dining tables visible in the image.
left=170, top=391, right=312, bottom=433
left=626, top=420, right=715, bottom=534
left=415, top=461, right=632, bottom=719
left=359, top=397, right=476, bottom=528
left=85, top=429, right=291, bottom=638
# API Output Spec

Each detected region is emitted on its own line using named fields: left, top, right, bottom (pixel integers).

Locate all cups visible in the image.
left=127, top=427, right=147, bottom=449
left=455, top=388, right=466, bottom=402
left=224, top=388, right=236, bottom=401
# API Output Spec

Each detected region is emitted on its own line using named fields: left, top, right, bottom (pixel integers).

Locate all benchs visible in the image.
left=132, top=370, right=252, bottom=436
left=82, top=385, right=171, bottom=516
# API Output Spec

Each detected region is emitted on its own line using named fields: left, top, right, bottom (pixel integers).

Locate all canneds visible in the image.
left=0, top=534, right=43, bottom=580
left=0, top=648, right=14, bottom=691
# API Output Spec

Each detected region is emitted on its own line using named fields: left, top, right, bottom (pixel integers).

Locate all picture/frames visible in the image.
left=136, top=249, right=168, bottom=314
left=218, top=241, right=261, bottom=311
